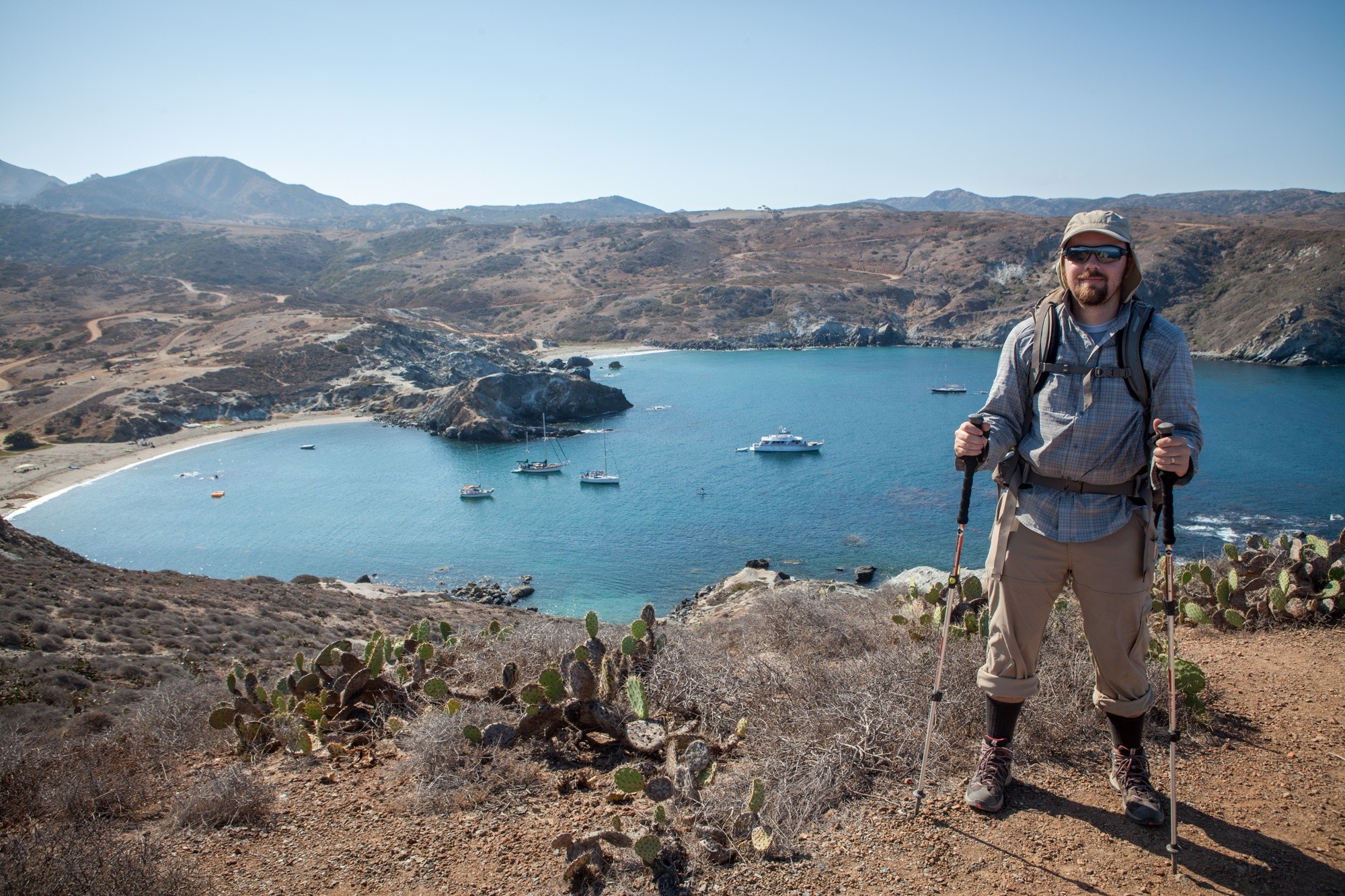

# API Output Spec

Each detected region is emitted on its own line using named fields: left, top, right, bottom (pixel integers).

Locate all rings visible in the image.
left=1171, top=457, right=1175, bottom=465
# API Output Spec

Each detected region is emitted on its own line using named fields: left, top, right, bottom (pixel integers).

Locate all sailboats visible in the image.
left=511, top=412, right=571, bottom=472
left=579, top=418, right=620, bottom=483
left=459, top=444, right=495, bottom=498
left=929, top=338, right=967, bottom=394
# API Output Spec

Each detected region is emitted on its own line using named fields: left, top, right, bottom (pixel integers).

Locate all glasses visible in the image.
left=1063, top=245, right=1127, bottom=264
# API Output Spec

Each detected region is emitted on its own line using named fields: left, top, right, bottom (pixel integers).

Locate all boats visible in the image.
left=212, top=491, right=225, bottom=498
left=299, top=444, right=316, bottom=450
left=735, top=425, right=825, bottom=453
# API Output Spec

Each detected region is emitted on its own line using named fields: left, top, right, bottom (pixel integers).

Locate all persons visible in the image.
left=953, top=210, right=1204, bottom=825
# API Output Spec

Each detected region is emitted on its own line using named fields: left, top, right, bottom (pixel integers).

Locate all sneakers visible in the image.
left=965, top=735, right=1014, bottom=812
left=1107, top=745, right=1165, bottom=825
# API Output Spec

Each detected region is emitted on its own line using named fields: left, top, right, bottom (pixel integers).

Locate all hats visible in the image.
left=1056, top=209, right=1143, bottom=303
